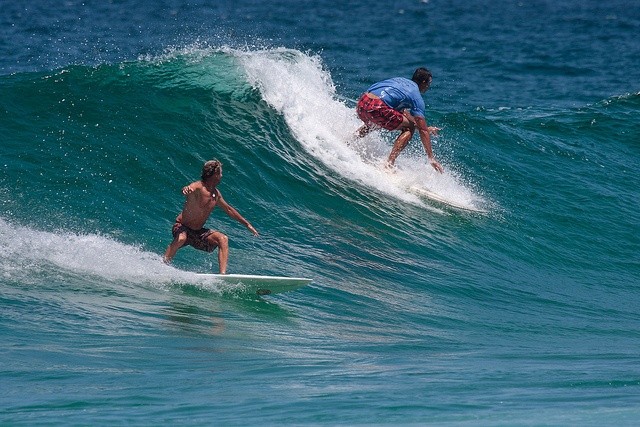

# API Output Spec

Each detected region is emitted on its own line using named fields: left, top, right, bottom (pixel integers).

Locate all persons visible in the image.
left=345, top=67, right=444, bottom=177
left=163, top=159, right=259, bottom=274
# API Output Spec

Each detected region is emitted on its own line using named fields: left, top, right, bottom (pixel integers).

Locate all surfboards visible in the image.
left=357, top=153, right=487, bottom=213
left=195, top=274, right=313, bottom=294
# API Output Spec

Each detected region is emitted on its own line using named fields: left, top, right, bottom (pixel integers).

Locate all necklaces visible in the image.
left=211, top=193, right=216, bottom=198
